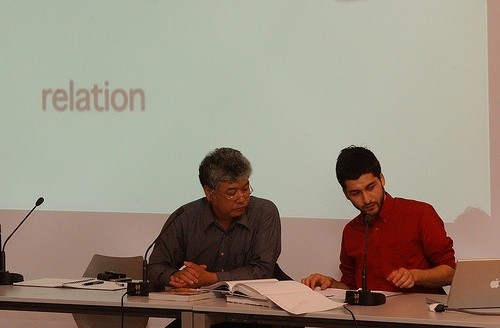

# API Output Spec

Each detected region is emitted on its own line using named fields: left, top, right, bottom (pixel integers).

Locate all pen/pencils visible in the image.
left=84, top=281, right=104, bottom=285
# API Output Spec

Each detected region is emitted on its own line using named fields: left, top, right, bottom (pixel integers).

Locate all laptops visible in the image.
left=426, top=259, right=500, bottom=309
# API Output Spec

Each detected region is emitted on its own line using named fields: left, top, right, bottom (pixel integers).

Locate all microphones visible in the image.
left=0, top=197, right=44, bottom=285
left=344, top=214, right=387, bottom=305
left=128, top=208, right=183, bottom=296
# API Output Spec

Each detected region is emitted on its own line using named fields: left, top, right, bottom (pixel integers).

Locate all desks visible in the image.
left=0, top=277, right=500, bottom=328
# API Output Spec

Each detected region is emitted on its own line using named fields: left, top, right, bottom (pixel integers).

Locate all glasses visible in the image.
left=217, top=185, right=255, bottom=202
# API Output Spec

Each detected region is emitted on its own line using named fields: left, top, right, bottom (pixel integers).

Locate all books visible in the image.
left=200, top=278, right=348, bottom=314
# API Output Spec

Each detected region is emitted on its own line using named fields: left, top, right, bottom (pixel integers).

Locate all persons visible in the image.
left=146, top=148, right=295, bottom=288
left=301, top=145, right=458, bottom=296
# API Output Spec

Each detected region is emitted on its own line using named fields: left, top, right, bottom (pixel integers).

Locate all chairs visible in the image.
left=70, top=254, right=149, bottom=328
